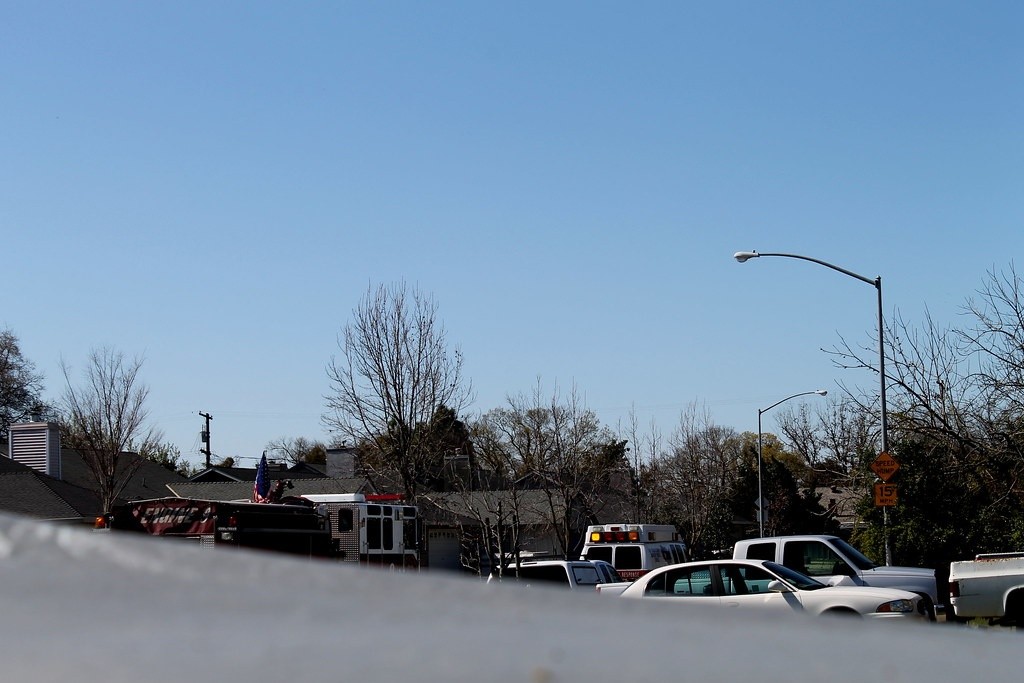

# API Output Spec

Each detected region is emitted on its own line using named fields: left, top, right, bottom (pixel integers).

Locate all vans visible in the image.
left=486, top=559, right=624, bottom=593
left=578, top=523, right=694, bottom=584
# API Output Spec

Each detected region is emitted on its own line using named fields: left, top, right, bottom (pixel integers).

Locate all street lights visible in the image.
left=758, top=389, right=830, bottom=537
left=734, top=251, right=896, bottom=566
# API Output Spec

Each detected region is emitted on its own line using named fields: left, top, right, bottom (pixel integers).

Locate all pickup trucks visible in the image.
left=949, top=552, right=1024, bottom=630
left=593, top=533, right=941, bottom=616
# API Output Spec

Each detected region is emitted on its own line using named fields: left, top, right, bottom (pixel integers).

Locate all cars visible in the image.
left=618, top=558, right=931, bottom=622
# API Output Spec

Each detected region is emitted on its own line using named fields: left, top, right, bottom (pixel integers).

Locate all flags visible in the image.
left=253, top=455, right=273, bottom=503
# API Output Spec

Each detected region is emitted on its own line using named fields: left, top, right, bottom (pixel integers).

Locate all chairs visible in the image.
left=703, top=584, right=714, bottom=595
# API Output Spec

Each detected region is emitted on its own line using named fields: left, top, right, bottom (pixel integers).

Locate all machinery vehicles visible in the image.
left=300, top=493, right=418, bottom=572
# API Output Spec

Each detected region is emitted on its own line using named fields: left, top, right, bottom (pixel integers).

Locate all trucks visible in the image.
left=89, top=476, right=347, bottom=561
